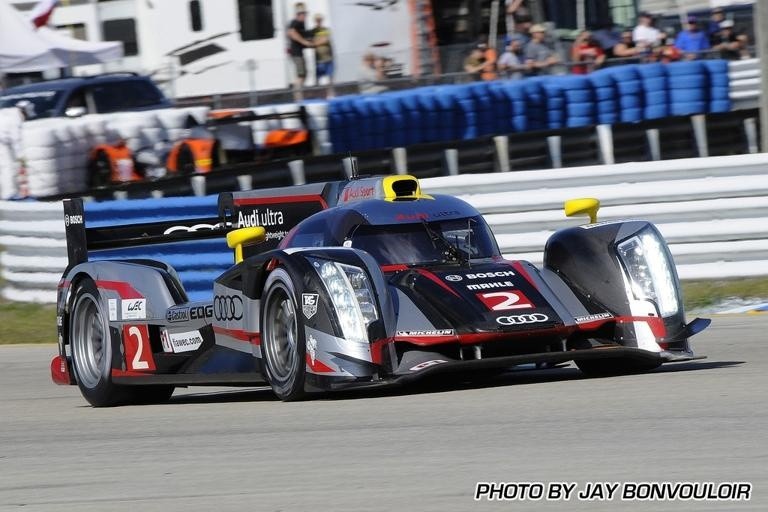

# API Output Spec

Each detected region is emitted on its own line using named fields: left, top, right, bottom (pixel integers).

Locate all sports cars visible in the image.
left=51, top=174, right=711, bottom=402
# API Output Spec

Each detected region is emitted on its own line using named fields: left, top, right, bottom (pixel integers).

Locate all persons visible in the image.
left=303, top=14, right=336, bottom=99
left=285, top=10, right=316, bottom=102
left=461, top=0, right=748, bottom=84
left=362, top=53, right=392, bottom=81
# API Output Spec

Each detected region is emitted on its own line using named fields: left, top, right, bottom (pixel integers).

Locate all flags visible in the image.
left=29, top=1, right=61, bottom=29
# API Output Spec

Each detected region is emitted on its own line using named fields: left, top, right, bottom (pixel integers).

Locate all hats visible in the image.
left=528, top=24, right=546, bottom=33
left=638, top=9, right=734, bottom=29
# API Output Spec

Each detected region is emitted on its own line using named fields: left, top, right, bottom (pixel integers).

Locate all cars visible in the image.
left=0, top=71, right=173, bottom=122
